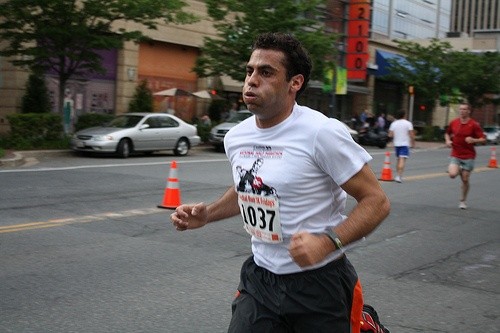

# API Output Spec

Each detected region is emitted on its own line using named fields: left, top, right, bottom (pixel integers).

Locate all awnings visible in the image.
left=206, top=57, right=324, bottom=92
left=373, top=49, right=442, bottom=85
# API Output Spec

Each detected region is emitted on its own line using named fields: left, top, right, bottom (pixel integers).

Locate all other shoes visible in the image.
left=395, top=177, right=402, bottom=183
left=459, top=200, right=466, bottom=208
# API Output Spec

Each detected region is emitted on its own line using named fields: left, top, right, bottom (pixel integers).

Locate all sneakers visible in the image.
left=360, top=304, right=390, bottom=333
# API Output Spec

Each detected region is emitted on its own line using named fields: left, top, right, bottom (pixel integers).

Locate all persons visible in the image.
left=387, top=109, right=417, bottom=184
left=357, top=109, right=396, bottom=137
left=200, top=113, right=214, bottom=126
left=443, top=102, right=486, bottom=209
left=229, top=102, right=239, bottom=119
left=220, top=106, right=230, bottom=123
left=61, top=88, right=75, bottom=135
left=169, top=31, right=390, bottom=333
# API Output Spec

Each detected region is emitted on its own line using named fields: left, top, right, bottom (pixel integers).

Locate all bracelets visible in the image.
left=324, top=229, right=345, bottom=250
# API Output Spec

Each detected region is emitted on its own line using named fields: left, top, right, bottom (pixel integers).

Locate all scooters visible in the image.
left=352, top=120, right=391, bottom=149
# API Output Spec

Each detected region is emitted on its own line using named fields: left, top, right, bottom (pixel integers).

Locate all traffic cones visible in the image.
left=376, top=151, right=396, bottom=181
left=487, top=146, right=500, bottom=168
left=156, top=160, right=183, bottom=210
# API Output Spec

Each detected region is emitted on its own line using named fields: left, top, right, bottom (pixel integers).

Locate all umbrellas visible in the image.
left=190, top=88, right=228, bottom=119
left=151, top=89, right=195, bottom=113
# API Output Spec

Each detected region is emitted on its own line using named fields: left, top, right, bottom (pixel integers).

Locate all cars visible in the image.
left=70, top=112, right=201, bottom=159
left=206, top=110, right=256, bottom=151
left=482, top=127, right=500, bottom=145
